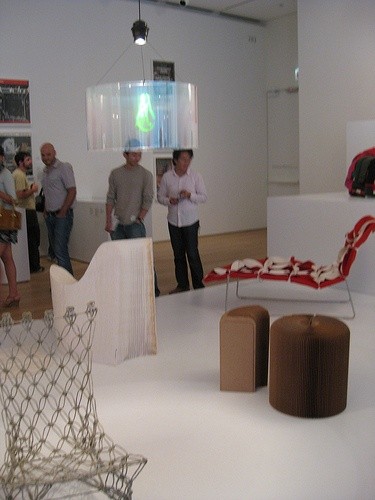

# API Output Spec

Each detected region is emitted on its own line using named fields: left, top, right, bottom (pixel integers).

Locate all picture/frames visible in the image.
left=149, top=55, right=177, bottom=102
left=0, top=131, right=35, bottom=180
left=154, top=154, right=174, bottom=201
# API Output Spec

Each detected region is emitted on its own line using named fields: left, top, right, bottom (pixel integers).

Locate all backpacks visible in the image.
left=345, top=148, right=375, bottom=196
left=349, top=156, right=375, bottom=199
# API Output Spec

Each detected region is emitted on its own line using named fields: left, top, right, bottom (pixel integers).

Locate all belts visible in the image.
left=46, top=208, right=73, bottom=215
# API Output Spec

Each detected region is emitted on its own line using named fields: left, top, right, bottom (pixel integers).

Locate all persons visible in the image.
left=40, top=143, right=76, bottom=276
left=0, top=145, right=20, bottom=307
left=105, top=139, right=160, bottom=297
left=157, top=148, right=207, bottom=294
left=12, top=152, right=44, bottom=272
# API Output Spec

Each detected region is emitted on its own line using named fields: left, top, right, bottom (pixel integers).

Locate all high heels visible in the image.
left=3, top=294, right=20, bottom=308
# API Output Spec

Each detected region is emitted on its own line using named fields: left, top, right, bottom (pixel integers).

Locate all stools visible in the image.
left=269, top=314, right=351, bottom=418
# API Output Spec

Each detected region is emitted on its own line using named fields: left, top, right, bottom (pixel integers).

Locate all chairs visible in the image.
left=204, top=215, right=375, bottom=319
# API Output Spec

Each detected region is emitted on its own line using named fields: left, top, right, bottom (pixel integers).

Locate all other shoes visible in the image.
left=36, top=266, right=45, bottom=273
left=168, top=283, right=190, bottom=294
left=193, top=281, right=206, bottom=289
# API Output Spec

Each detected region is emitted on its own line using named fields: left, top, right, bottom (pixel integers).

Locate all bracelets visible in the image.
left=137, top=216, right=144, bottom=223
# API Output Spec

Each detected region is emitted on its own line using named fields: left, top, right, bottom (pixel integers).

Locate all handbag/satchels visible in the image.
left=35, top=186, right=45, bottom=212
left=0, top=198, right=21, bottom=231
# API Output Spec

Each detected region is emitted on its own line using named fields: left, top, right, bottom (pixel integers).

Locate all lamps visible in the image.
left=131, top=0, right=149, bottom=46
left=85, top=42, right=201, bottom=153
